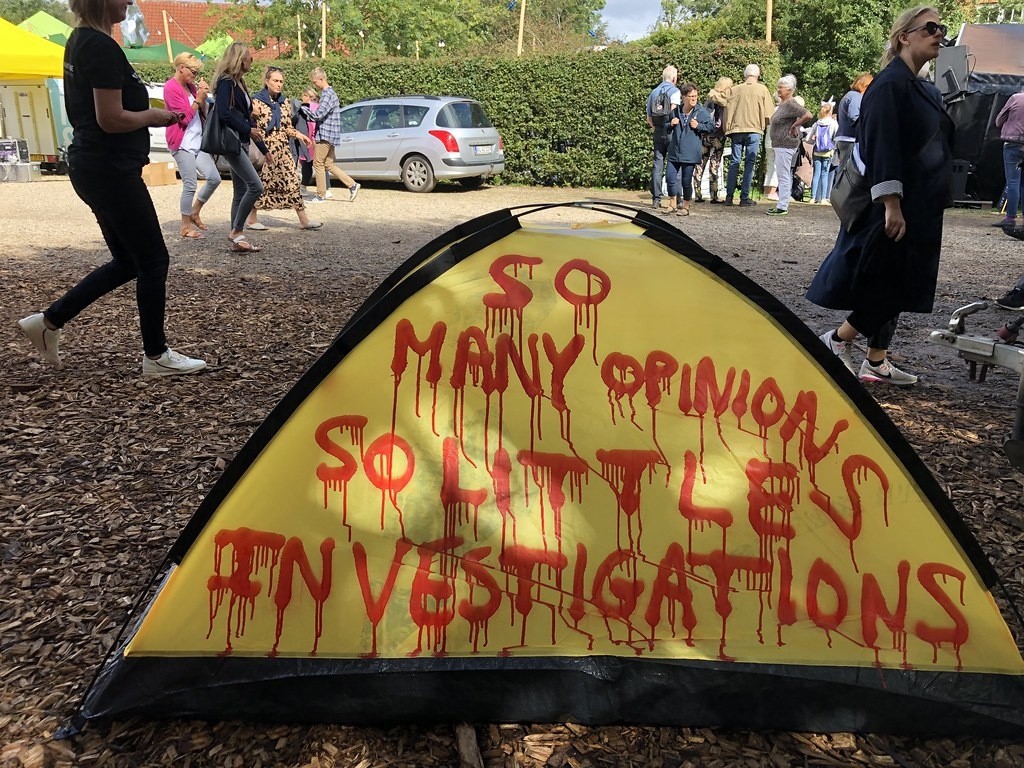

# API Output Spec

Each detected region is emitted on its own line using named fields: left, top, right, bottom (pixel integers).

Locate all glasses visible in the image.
left=185, top=66, right=198, bottom=76
left=266, top=67, right=283, bottom=79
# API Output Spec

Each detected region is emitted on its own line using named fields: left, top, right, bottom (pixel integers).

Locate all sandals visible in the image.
left=662, top=205, right=690, bottom=215
left=179, top=214, right=209, bottom=240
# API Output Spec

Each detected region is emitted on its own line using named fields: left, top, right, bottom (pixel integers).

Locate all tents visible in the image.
left=123, top=37, right=210, bottom=67
left=15, top=10, right=74, bottom=50
left=51, top=204, right=1024, bottom=750
left=0, top=14, right=67, bottom=79
left=192, top=30, right=236, bottom=59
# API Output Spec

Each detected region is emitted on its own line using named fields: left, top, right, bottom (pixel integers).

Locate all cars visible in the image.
left=310, top=94, right=505, bottom=193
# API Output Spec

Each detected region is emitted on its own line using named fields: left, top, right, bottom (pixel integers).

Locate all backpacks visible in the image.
left=652, top=85, right=674, bottom=127
left=813, top=120, right=837, bottom=153
left=701, top=99, right=724, bottom=140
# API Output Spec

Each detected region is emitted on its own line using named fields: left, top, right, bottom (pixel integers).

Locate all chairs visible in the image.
left=369, top=109, right=388, bottom=129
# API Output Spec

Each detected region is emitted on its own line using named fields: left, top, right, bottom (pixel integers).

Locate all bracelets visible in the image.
left=194, top=101, right=200, bottom=109
left=793, top=124, right=797, bottom=128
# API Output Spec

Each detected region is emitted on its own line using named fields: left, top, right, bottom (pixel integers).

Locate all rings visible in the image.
left=890, top=227, right=894, bottom=229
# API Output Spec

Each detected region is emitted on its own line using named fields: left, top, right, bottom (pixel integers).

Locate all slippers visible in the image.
left=296, top=221, right=324, bottom=230
left=246, top=223, right=269, bottom=230
left=227, top=234, right=262, bottom=252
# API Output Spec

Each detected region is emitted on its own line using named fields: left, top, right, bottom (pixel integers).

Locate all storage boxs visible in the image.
left=142, top=161, right=177, bottom=186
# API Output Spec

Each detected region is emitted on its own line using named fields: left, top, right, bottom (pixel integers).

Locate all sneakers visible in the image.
left=805, top=199, right=832, bottom=207
left=300, top=185, right=308, bottom=197
left=739, top=196, right=757, bottom=206
left=765, top=207, right=788, bottom=216
left=993, top=282, right=1023, bottom=310
left=17, top=312, right=62, bottom=370
left=990, top=218, right=1024, bottom=243
left=819, top=329, right=856, bottom=377
left=724, top=196, right=733, bottom=205
left=303, top=188, right=333, bottom=203
left=348, top=182, right=361, bottom=202
left=142, top=347, right=207, bottom=379
left=859, top=358, right=918, bottom=384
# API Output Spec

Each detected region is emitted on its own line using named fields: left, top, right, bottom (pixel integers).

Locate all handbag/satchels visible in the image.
left=830, top=139, right=873, bottom=234
left=213, top=138, right=267, bottom=172
left=200, top=75, right=241, bottom=157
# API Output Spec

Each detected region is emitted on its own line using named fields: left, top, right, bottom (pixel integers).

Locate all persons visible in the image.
left=18, top=1, right=207, bottom=379
left=247, top=65, right=326, bottom=230
left=707, top=63, right=776, bottom=206
left=991, top=79, right=1024, bottom=227
left=818, top=8, right=957, bottom=385
left=211, top=41, right=276, bottom=254
left=163, top=50, right=223, bottom=238
left=646, top=67, right=681, bottom=210
left=662, top=84, right=714, bottom=216
left=297, top=66, right=360, bottom=202
left=765, top=76, right=813, bottom=216
left=699, top=77, right=733, bottom=204
left=764, top=72, right=875, bottom=205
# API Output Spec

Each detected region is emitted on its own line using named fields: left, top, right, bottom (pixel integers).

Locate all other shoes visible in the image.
left=763, top=192, right=780, bottom=201
left=710, top=197, right=723, bottom=204
left=677, top=202, right=685, bottom=209
left=651, top=199, right=661, bottom=210
left=694, top=197, right=705, bottom=203
